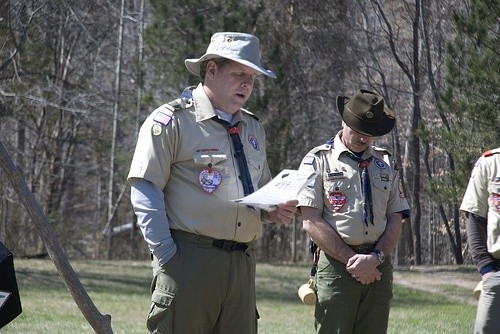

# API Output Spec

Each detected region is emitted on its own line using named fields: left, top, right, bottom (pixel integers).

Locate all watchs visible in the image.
left=372, top=250, right=385, bottom=264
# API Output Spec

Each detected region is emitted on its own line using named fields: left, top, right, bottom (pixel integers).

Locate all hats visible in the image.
left=336, top=89, right=397, bottom=138
left=185, top=32, right=278, bottom=81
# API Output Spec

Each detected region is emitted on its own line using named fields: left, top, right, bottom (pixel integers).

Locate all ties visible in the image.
left=212, top=115, right=254, bottom=198
left=344, top=151, right=375, bottom=226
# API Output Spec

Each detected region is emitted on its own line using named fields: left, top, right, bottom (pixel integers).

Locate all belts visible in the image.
left=348, top=243, right=376, bottom=253
left=173, top=230, right=246, bottom=251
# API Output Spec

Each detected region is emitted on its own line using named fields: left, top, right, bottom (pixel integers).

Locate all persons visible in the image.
left=127, top=32, right=299, bottom=334
left=459, top=147, right=500, bottom=334
left=296, top=89, right=410, bottom=334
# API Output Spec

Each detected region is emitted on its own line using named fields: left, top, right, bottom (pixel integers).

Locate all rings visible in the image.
left=354, top=274, right=356, bottom=277
